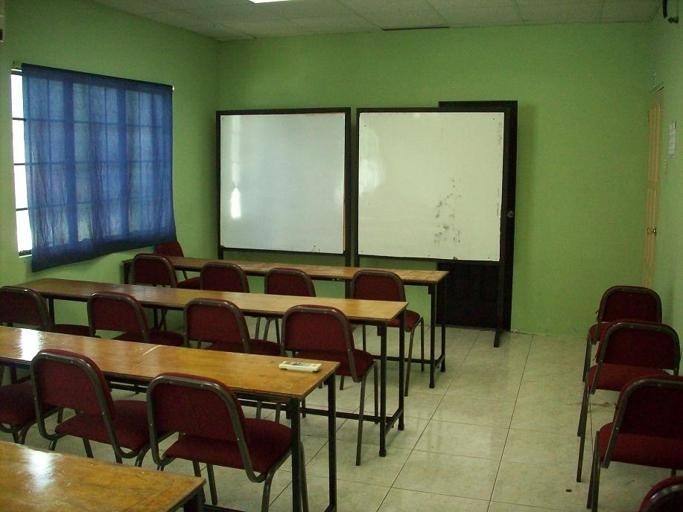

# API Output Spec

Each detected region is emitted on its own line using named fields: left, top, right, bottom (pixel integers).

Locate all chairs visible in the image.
left=576, top=285, right=682, bottom=512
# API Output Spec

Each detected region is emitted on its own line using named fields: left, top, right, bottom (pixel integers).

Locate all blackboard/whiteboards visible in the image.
left=354, top=107, right=511, bottom=266
left=216, top=107, right=351, bottom=256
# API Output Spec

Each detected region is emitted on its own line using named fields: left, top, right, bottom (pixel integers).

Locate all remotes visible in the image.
left=279, top=361, right=322, bottom=373
